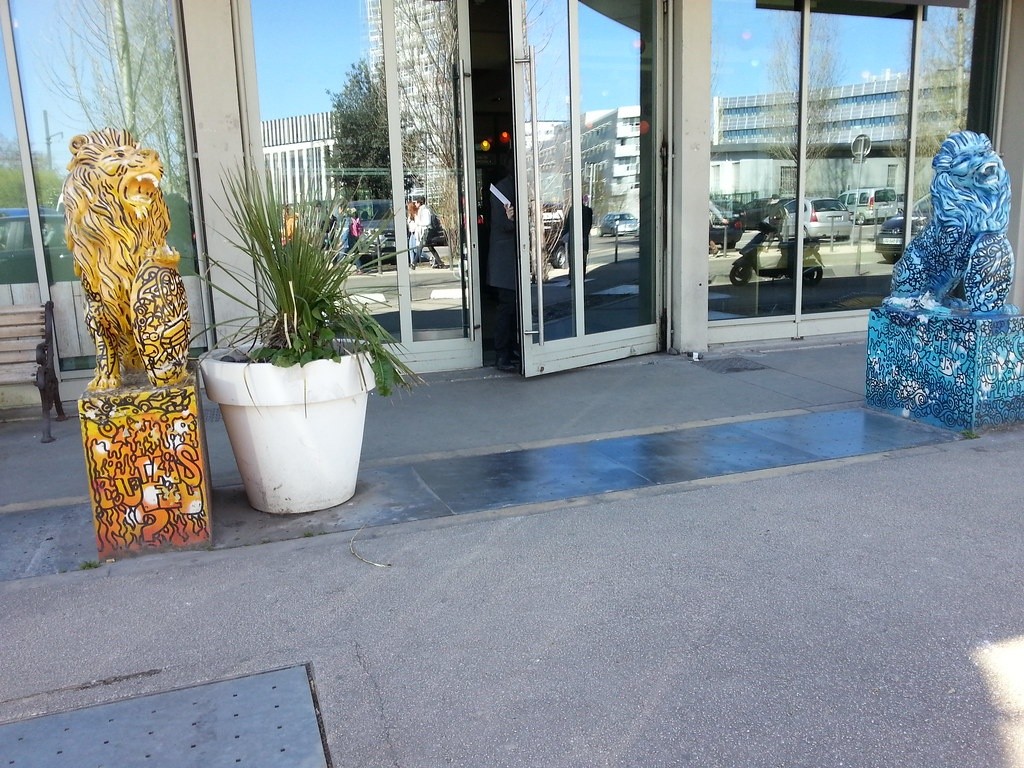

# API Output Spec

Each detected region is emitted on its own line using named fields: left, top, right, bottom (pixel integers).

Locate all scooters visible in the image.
left=729, top=218, right=825, bottom=287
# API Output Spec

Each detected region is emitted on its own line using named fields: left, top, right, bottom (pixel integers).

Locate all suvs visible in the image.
left=875, top=191, right=932, bottom=264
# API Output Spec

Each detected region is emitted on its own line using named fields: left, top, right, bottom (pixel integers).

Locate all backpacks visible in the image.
left=351, top=218, right=362, bottom=237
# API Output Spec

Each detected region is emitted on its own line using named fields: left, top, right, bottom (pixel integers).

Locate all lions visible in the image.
left=63, top=127, right=196, bottom=391
left=890, top=131, right=1012, bottom=310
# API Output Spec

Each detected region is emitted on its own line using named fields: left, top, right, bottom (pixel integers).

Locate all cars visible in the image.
left=431, top=223, right=449, bottom=247
left=709, top=198, right=772, bottom=249
left=347, top=200, right=411, bottom=268
left=541, top=203, right=567, bottom=269
left=597, top=212, right=639, bottom=238
left=897, top=192, right=905, bottom=218
left=762, top=197, right=853, bottom=244
left=0, top=208, right=81, bottom=283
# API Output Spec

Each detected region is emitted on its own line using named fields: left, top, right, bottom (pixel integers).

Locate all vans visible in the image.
left=835, top=187, right=898, bottom=226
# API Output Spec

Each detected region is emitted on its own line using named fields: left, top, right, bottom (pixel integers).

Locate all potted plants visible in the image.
left=195, top=155, right=426, bottom=514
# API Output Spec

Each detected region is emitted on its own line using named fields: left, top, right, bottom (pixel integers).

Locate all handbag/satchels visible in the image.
left=431, top=215, right=439, bottom=229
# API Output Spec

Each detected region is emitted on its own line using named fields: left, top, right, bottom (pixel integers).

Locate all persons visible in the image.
left=763, top=194, right=783, bottom=253
left=710, top=200, right=730, bottom=256
left=407, top=196, right=444, bottom=269
left=280, top=200, right=364, bottom=274
left=559, top=200, right=593, bottom=286
left=485, top=148, right=520, bottom=370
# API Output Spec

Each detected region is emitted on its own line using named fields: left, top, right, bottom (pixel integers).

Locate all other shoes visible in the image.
left=510, top=353, right=520, bottom=363
left=433, top=263, right=443, bottom=269
left=429, top=257, right=434, bottom=267
left=496, top=356, right=516, bottom=371
left=409, top=263, right=416, bottom=270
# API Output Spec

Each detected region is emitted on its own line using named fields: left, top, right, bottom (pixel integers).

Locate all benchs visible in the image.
left=0, top=300, right=70, bottom=443
